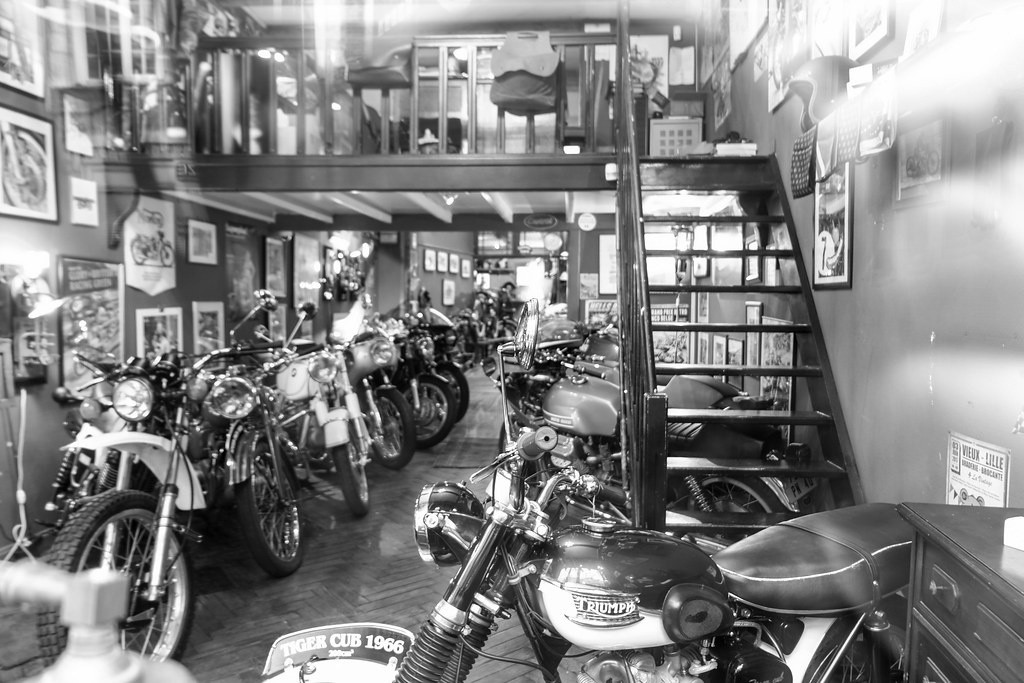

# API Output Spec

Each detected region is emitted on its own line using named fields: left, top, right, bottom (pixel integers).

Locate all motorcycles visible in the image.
left=256, top=299, right=918, bottom=683
left=31, top=288, right=470, bottom=680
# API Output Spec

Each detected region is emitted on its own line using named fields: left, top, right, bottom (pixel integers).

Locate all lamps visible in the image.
left=166, top=110, right=188, bottom=138
left=11, top=270, right=68, bottom=320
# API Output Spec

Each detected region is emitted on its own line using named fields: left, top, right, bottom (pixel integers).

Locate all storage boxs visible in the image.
left=648, top=118, right=702, bottom=157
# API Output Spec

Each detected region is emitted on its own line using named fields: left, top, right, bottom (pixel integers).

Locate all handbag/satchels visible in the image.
left=490, top=30, right=561, bottom=111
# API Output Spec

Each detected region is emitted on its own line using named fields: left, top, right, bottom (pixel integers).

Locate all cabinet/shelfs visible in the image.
left=895, top=503, right=1024, bottom=683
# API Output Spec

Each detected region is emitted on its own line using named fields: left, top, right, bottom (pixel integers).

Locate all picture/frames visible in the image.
left=185, top=218, right=218, bottom=267
left=264, top=236, right=287, bottom=300
left=743, top=299, right=763, bottom=379
left=891, top=100, right=953, bottom=209
left=192, top=300, right=226, bottom=371
left=726, top=337, right=744, bottom=393
left=711, top=333, right=729, bottom=386
left=424, top=249, right=472, bottom=306
left=0, top=0, right=45, bottom=101
left=759, top=316, right=798, bottom=446
left=0, top=102, right=60, bottom=223
left=55, top=254, right=125, bottom=405
left=810, top=157, right=852, bottom=289
left=847, top=0, right=896, bottom=66
left=744, top=234, right=761, bottom=284
left=768, top=0, right=815, bottom=112
left=136, top=306, right=184, bottom=366
